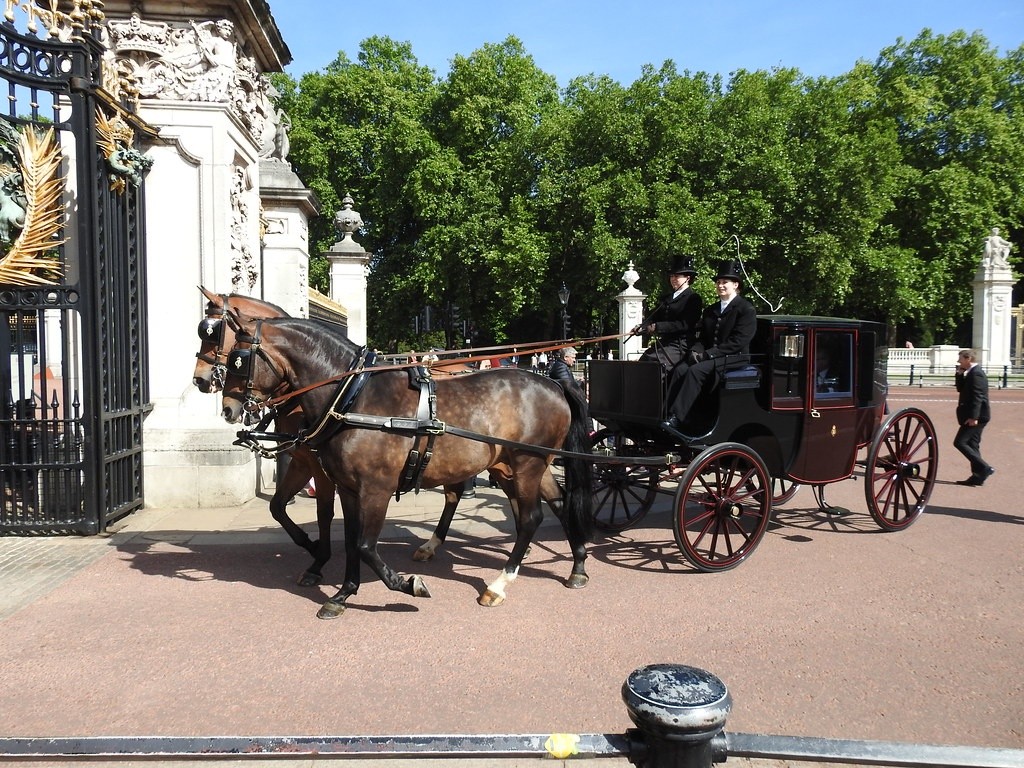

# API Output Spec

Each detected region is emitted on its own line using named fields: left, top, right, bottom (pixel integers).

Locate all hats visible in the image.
left=665, top=255, right=698, bottom=276
left=712, top=263, right=740, bottom=284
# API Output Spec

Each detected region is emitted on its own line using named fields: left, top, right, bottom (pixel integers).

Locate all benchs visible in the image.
left=691, top=329, right=773, bottom=394
left=769, top=369, right=850, bottom=403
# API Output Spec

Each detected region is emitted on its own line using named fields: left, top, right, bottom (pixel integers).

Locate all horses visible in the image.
left=224, top=308, right=593, bottom=620
left=192, top=285, right=533, bottom=563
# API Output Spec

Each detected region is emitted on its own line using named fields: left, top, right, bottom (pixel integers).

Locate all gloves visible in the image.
left=689, top=350, right=704, bottom=364
left=644, top=323, right=656, bottom=336
left=632, top=324, right=644, bottom=336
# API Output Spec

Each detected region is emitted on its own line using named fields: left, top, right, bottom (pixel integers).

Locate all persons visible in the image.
left=632, top=261, right=702, bottom=383
left=816, top=349, right=838, bottom=393
left=388, top=339, right=397, bottom=353
left=953, top=350, right=995, bottom=487
left=664, top=260, right=757, bottom=428
left=421, top=348, right=438, bottom=375
left=408, top=350, right=419, bottom=363
left=511, top=347, right=519, bottom=368
left=550, top=347, right=578, bottom=380
left=530, top=343, right=561, bottom=375
left=585, top=345, right=613, bottom=367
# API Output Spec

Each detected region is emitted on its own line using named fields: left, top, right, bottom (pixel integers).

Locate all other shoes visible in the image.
left=956, top=477, right=981, bottom=485
left=664, top=414, right=679, bottom=429
left=977, top=463, right=993, bottom=484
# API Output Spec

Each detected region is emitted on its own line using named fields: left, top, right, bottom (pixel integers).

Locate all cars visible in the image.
left=472, top=357, right=518, bottom=369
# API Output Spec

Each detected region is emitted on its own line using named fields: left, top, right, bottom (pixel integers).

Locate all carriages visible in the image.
left=192, top=284, right=940, bottom=620
